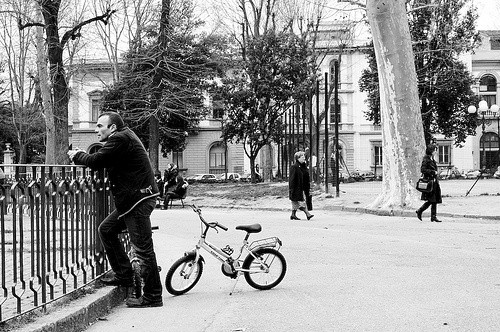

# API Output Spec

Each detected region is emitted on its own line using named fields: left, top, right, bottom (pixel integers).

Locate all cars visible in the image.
left=437, top=169, right=461, bottom=180
left=493, top=166, right=500, bottom=179
left=464, top=169, right=491, bottom=180
left=184, top=170, right=375, bottom=182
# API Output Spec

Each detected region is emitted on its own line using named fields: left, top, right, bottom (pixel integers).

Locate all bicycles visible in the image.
left=165, top=203, right=287, bottom=296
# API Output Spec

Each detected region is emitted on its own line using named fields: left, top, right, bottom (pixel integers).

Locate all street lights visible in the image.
left=468, top=100, right=499, bottom=179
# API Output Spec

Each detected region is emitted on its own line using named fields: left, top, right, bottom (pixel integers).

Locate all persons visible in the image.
left=0, top=167, right=5, bottom=198
left=306, top=148, right=317, bottom=183
left=154, top=162, right=189, bottom=210
left=331, top=152, right=336, bottom=187
left=440, top=167, right=461, bottom=179
left=255, top=164, right=262, bottom=183
left=415, top=144, right=443, bottom=222
left=494, top=166, right=500, bottom=178
left=67, top=111, right=163, bottom=308
left=289, top=151, right=314, bottom=221
left=293, top=142, right=309, bottom=206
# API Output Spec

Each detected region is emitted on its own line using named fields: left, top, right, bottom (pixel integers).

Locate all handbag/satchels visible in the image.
left=416, top=177, right=433, bottom=192
left=306, top=194, right=312, bottom=211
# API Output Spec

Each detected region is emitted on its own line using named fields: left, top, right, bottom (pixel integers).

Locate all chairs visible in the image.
left=156, top=185, right=186, bottom=209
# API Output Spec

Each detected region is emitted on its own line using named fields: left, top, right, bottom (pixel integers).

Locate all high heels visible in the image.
left=416, top=210, right=422, bottom=221
left=307, top=215, right=313, bottom=220
left=431, top=217, right=442, bottom=222
left=291, top=215, right=300, bottom=220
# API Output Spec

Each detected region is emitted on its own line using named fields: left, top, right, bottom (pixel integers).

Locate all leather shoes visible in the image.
left=126, top=296, right=163, bottom=308
left=100, top=276, right=136, bottom=287
